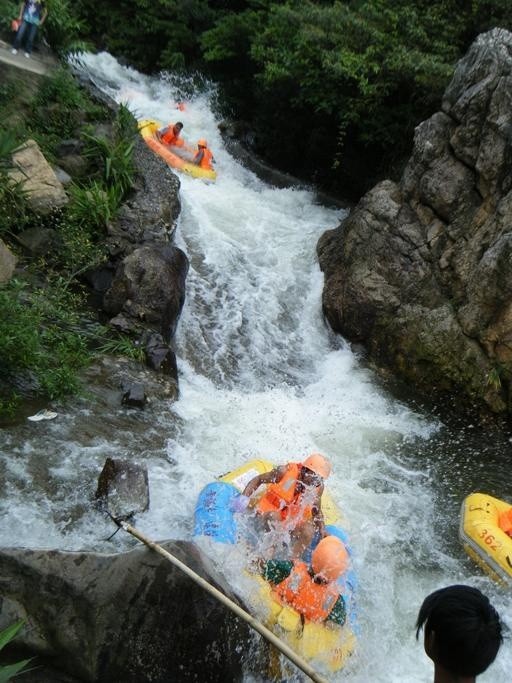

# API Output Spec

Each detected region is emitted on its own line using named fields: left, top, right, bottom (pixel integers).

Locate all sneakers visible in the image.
left=25, top=53, right=30, bottom=59
left=11, top=48, right=17, bottom=55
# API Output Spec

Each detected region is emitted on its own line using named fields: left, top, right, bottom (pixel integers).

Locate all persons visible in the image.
left=413, top=585, right=504, bottom=683
left=12, top=1, right=48, bottom=58
left=157, top=121, right=183, bottom=146
left=252, top=535, right=350, bottom=632
left=191, top=138, right=216, bottom=169
left=176, top=98, right=185, bottom=111
left=242, top=452, right=333, bottom=561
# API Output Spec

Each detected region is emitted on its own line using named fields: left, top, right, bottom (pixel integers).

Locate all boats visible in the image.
left=457, top=493, right=512, bottom=592
left=195, top=460, right=360, bottom=679
left=138, top=118, right=217, bottom=182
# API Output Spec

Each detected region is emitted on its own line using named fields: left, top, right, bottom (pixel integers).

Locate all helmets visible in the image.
left=312, top=536, right=348, bottom=582
left=198, top=138, right=207, bottom=148
left=303, top=454, right=330, bottom=478
